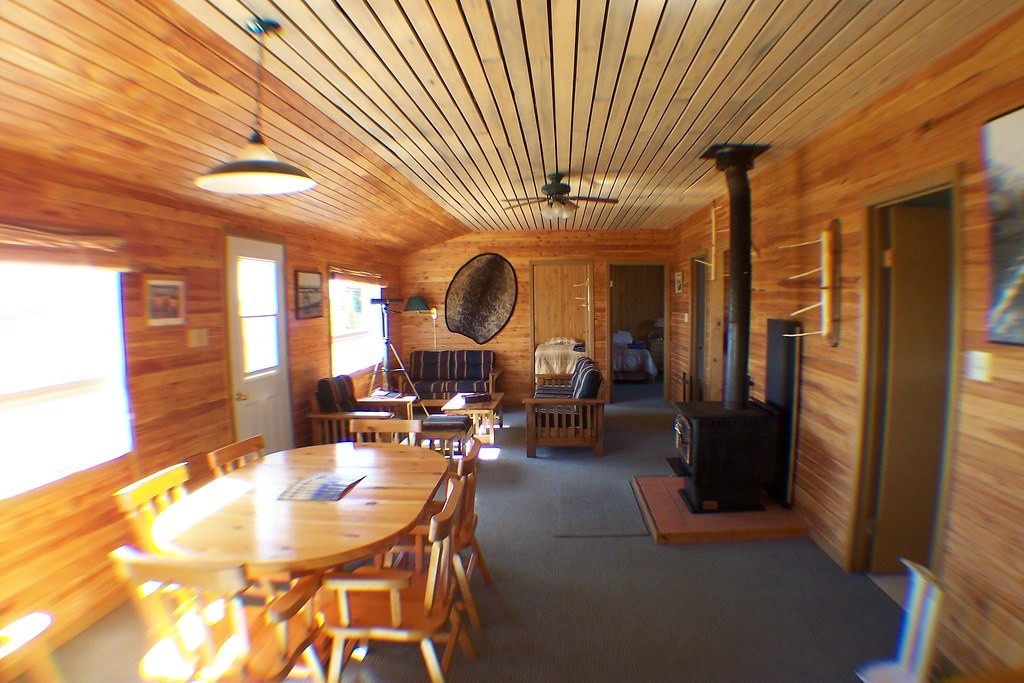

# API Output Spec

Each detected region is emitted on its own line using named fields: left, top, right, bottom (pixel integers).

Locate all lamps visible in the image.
left=653, top=317, right=665, bottom=328
left=192, top=16, right=318, bottom=195
left=542, top=195, right=579, bottom=220
left=404, top=295, right=438, bottom=352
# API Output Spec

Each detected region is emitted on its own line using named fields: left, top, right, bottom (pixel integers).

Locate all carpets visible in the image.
left=554, top=479, right=650, bottom=536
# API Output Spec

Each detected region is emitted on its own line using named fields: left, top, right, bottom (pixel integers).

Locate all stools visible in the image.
left=416, top=414, right=474, bottom=459
left=0, top=609, right=65, bottom=683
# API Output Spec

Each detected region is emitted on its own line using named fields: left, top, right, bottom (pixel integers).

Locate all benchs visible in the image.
left=520, top=355, right=605, bottom=457
left=391, top=350, right=505, bottom=408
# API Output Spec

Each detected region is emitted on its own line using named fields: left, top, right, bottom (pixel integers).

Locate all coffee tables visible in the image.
left=440, top=392, right=506, bottom=446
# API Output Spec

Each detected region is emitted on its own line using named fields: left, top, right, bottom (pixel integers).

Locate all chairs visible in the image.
left=310, top=373, right=410, bottom=445
left=109, top=416, right=492, bottom=683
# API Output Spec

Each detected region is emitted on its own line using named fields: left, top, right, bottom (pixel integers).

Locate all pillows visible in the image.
left=612, top=330, right=634, bottom=345
left=545, top=337, right=577, bottom=346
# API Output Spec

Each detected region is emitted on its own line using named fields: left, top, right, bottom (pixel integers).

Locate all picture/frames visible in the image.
left=674, top=271, right=682, bottom=294
left=140, top=272, right=189, bottom=332
left=293, top=269, right=324, bottom=320
left=979, top=104, right=1023, bottom=345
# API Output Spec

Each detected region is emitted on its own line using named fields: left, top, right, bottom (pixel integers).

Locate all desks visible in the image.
left=150, top=441, right=450, bottom=667
left=357, top=396, right=417, bottom=419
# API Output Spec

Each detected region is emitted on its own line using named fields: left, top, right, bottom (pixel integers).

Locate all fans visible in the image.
left=501, top=173, right=619, bottom=213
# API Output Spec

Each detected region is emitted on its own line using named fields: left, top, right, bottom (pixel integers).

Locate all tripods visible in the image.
left=367, top=305, right=430, bottom=418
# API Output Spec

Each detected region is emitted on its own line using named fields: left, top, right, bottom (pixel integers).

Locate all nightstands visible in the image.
left=639, top=337, right=665, bottom=372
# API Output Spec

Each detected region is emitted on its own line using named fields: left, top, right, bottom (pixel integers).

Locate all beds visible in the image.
left=534, top=343, right=586, bottom=374
left=610, top=342, right=658, bottom=384
